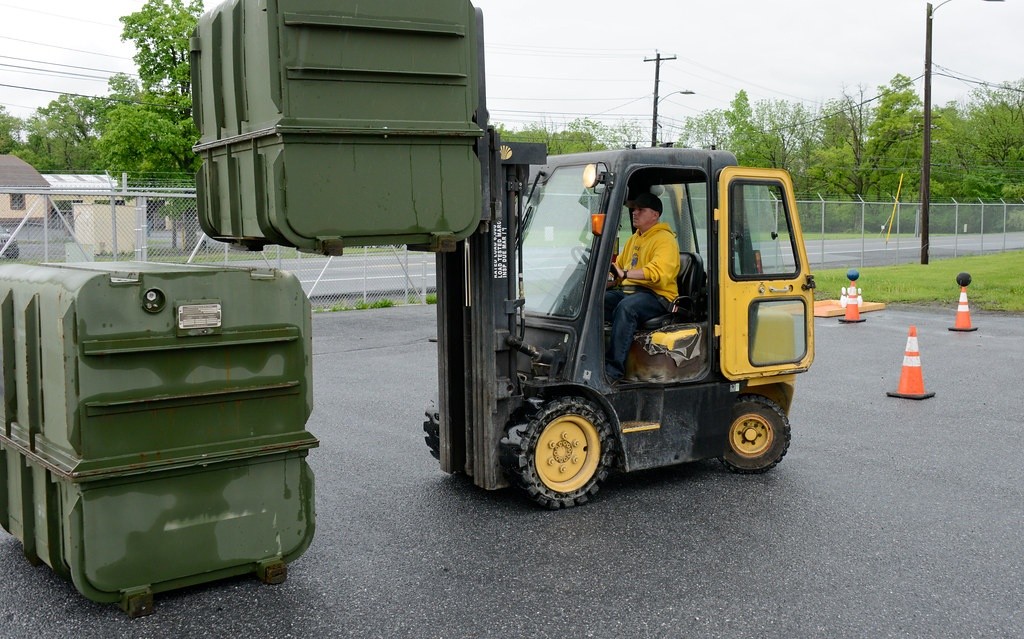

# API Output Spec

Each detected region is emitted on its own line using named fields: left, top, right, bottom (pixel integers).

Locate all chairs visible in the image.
left=642, top=250, right=703, bottom=334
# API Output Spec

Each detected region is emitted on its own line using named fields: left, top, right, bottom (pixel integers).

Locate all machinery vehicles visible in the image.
left=422, top=6, right=816, bottom=510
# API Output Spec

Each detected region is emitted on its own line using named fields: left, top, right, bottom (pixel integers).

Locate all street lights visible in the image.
left=651, top=90, right=695, bottom=148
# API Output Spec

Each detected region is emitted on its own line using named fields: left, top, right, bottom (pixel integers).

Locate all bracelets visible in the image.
left=623, top=269, right=628, bottom=278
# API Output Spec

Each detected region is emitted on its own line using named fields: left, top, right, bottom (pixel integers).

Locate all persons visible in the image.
left=603, top=192, right=680, bottom=385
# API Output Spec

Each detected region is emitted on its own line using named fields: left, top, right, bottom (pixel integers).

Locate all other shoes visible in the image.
left=606, top=374, right=616, bottom=385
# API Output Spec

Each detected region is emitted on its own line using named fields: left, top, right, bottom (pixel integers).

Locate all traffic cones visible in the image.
left=839, top=281, right=866, bottom=322
left=886, top=325, right=936, bottom=399
left=947, top=286, right=979, bottom=331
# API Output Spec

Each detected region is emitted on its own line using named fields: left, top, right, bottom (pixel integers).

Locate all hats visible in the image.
left=622, top=192, right=662, bottom=217
